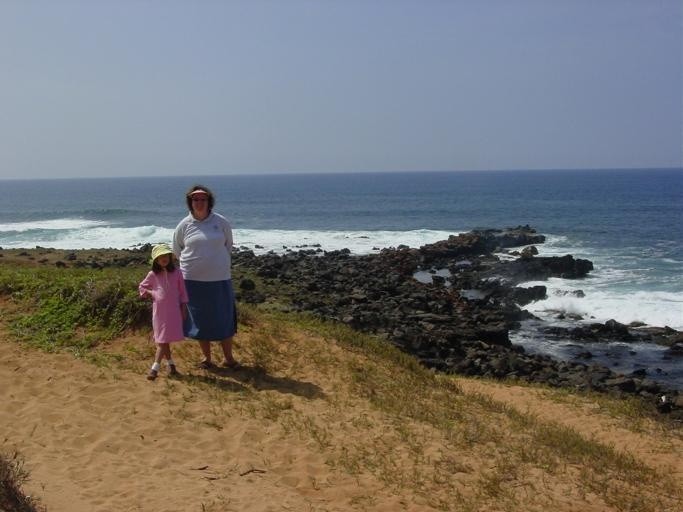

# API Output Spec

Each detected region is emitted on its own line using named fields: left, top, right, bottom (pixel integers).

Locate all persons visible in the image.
left=138, top=243, right=190, bottom=381
left=171, top=184, right=242, bottom=371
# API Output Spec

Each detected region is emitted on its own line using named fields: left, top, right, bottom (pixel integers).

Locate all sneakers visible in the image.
left=146, top=370, right=158, bottom=379
left=165, top=364, right=176, bottom=374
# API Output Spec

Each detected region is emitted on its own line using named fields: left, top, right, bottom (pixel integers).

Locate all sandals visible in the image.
left=200, top=361, right=212, bottom=369
left=221, top=360, right=241, bottom=370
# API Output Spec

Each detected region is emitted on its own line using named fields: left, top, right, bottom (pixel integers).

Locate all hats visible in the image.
left=187, top=188, right=207, bottom=199
left=151, top=243, right=176, bottom=266
